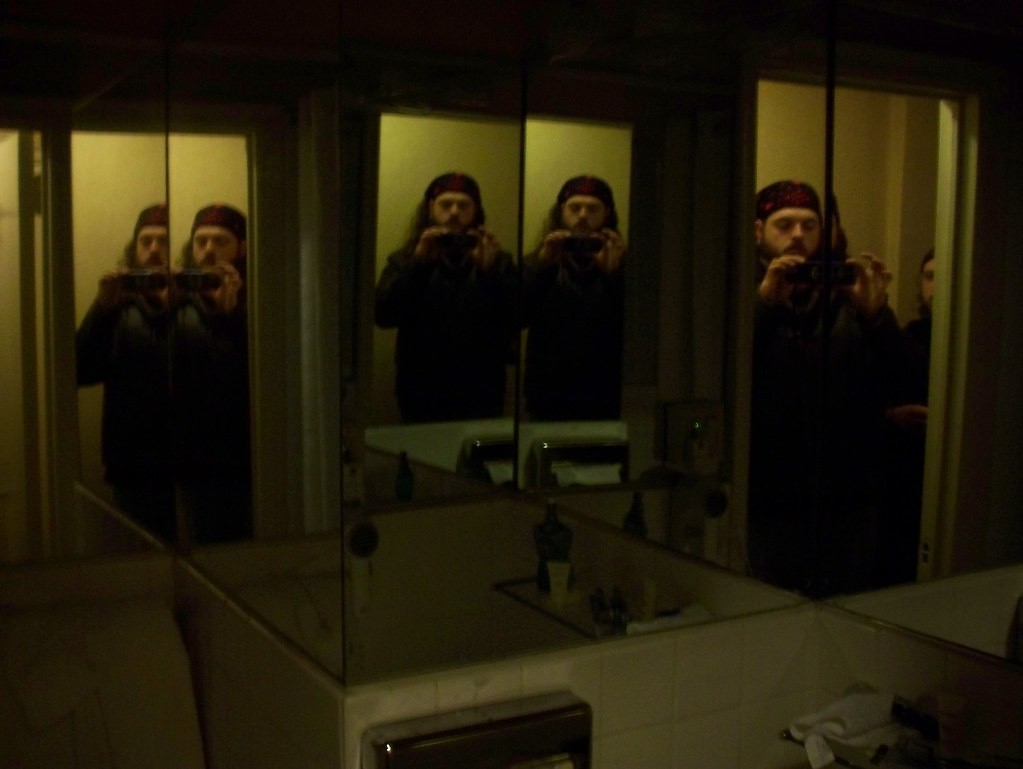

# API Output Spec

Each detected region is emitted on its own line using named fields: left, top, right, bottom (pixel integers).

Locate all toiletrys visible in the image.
left=532, top=504, right=633, bottom=628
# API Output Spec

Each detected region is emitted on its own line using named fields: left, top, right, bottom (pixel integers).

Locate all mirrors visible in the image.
left=71, top=0, right=1023, bottom=689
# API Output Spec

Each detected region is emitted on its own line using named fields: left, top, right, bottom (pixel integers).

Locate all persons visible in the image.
left=372, top=173, right=519, bottom=425
left=169, top=205, right=253, bottom=545
left=74, top=208, right=169, bottom=541
left=748, top=182, right=934, bottom=599
left=522, top=176, right=628, bottom=420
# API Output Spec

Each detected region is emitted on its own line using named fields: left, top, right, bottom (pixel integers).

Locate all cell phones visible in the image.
left=561, top=236, right=603, bottom=254
left=175, top=269, right=222, bottom=291
left=120, top=267, right=165, bottom=292
left=436, top=230, right=478, bottom=248
left=794, top=259, right=859, bottom=285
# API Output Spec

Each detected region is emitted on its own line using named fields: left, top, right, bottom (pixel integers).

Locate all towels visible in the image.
left=789, top=693, right=896, bottom=741
